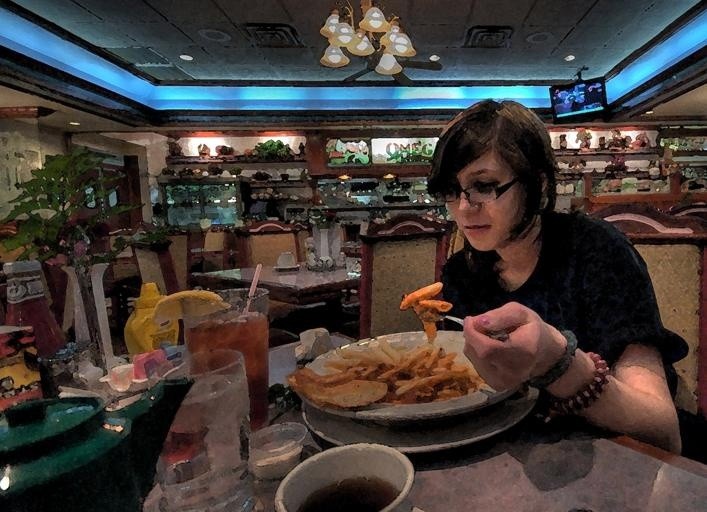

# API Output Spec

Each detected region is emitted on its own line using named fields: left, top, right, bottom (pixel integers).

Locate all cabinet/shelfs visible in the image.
left=157, top=146, right=707, bottom=213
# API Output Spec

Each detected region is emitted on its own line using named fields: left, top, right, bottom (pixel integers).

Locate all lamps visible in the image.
left=318, top=3, right=418, bottom=76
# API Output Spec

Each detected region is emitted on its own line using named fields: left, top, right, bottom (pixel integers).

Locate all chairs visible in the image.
left=1, top=197, right=707, bottom=463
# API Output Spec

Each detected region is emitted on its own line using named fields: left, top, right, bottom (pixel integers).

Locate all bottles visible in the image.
left=3, top=260, right=67, bottom=355
left=123, top=283, right=180, bottom=357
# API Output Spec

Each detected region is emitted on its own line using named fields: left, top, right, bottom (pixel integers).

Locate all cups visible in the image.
left=278, top=252, right=296, bottom=266
left=274, top=442, right=416, bottom=511
left=157, top=286, right=270, bottom=512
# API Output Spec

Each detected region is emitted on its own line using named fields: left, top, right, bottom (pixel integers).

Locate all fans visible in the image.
left=343, top=41, right=442, bottom=87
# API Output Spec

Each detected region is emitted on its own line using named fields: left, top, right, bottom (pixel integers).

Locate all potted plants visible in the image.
left=1, top=145, right=168, bottom=376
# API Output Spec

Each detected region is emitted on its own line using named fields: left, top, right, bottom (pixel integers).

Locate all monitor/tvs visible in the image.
left=550, top=76, right=607, bottom=124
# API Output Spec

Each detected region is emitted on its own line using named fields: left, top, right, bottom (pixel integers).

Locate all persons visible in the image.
left=427, top=97, right=683, bottom=456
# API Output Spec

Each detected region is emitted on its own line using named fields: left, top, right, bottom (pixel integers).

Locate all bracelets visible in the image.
left=530, top=327, right=579, bottom=390
left=544, top=351, right=610, bottom=419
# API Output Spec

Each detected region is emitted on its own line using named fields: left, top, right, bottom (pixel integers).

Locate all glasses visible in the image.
left=433, top=176, right=519, bottom=205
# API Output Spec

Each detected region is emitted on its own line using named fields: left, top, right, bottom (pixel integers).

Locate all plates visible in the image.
left=304, top=332, right=540, bottom=457
left=273, top=264, right=300, bottom=271
